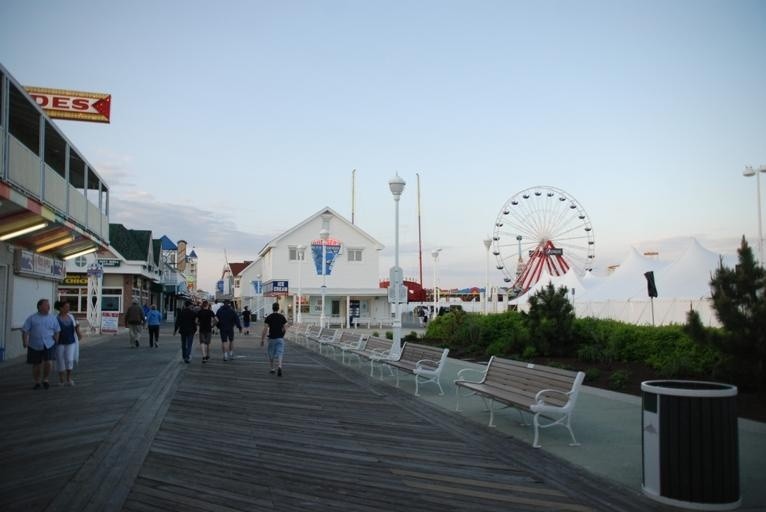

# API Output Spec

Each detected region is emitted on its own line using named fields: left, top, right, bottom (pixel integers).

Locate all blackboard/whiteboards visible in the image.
left=640, top=380, right=742, bottom=511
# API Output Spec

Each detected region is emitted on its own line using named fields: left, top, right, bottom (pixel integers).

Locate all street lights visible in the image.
left=247, top=277, right=252, bottom=314
left=429, top=246, right=443, bottom=321
left=386, top=170, right=408, bottom=332
left=255, top=271, right=262, bottom=321
left=318, top=227, right=330, bottom=327
left=482, top=233, right=492, bottom=317
left=740, top=155, right=765, bottom=269
left=294, top=241, right=306, bottom=322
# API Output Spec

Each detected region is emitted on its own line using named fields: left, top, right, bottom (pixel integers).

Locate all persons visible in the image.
left=261, top=302, right=287, bottom=376
left=141, top=302, right=151, bottom=330
left=124, top=297, right=145, bottom=349
left=173, top=298, right=251, bottom=363
left=418, top=307, right=428, bottom=328
left=144, top=304, right=162, bottom=348
left=53, top=300, right=82, bottom=387
left=21, top=299, right=62, bottom=389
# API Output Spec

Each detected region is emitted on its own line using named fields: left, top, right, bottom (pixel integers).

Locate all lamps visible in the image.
left=0, top=222, right=50, bottom=242
left=62, top=245, right=99, bottom=261
left=35, top=235, right=76, bottom=254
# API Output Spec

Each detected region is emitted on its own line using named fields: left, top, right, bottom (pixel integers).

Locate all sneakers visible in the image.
left=32, top=380, right=74, bottom=390
left=184, top=353, right=236, bottom=364
left=270, top=366, right=282, bottom=376
left=130, top=339, right=140, bottom=349
left=150, top=341, right=159, bottom=348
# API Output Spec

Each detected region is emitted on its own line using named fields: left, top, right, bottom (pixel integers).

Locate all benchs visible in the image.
left=346, top=334, right=396, bottom=380
left=320, top=316, right=392, bottom=329
left=450, top=355, right=586, bottom=450
left=284, top=321, right=322, bottom=348
left=323, top=331, right=364, bottom=365
left=305, top=327, right=340, bottom=354
left=375, top=341, right=450, bottom=398
